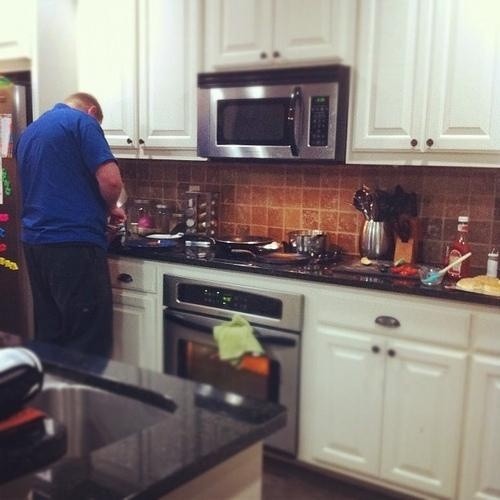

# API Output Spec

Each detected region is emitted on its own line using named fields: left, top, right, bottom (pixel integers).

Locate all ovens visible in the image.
left=161, top=308, right=296, bottom=458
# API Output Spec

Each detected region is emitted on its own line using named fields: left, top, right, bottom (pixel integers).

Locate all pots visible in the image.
left=288, top=228, right=329, bottom=257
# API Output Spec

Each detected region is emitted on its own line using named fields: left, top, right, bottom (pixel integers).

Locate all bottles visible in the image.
left=124, top=197, right=185, bottom=238
left=486, top=253, right=499, bottom=278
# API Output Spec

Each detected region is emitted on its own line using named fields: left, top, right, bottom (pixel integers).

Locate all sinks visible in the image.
left=26, top=374, right=179, bottom=475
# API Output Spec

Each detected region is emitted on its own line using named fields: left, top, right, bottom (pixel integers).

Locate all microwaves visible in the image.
left=195, top=64, right=350, bottom=163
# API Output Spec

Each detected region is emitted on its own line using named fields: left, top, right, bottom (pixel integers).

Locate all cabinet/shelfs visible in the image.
left=300, top=282, right=474, bottom=500
left=69, top=0, right=208, bottom=161
left=345, top=0, right=499, bottom=169
left=203, top=0, right=356, bottom=74
left=106, top=251, right=163, bottom=376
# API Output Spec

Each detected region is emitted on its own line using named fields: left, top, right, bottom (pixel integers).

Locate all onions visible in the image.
left=138, top=208, right=155, bottom=228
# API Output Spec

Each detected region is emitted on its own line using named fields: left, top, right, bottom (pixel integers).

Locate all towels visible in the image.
left=213, top=315, right=264, bottom=368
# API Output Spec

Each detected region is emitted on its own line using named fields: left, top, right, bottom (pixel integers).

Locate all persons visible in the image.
left=16, top=95, right=127, bottom=356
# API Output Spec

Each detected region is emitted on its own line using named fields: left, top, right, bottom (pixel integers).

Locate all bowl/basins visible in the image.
left=146, top=233, right=180, bottom=247
left=418, top=267, right=445, bottom=286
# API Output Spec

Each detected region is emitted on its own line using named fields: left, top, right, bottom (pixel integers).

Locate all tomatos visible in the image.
left=391, top=266, right=418, bottom=277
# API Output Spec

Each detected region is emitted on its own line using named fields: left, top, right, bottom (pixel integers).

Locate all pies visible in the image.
left=455, top=273, right=500, bottom=292
